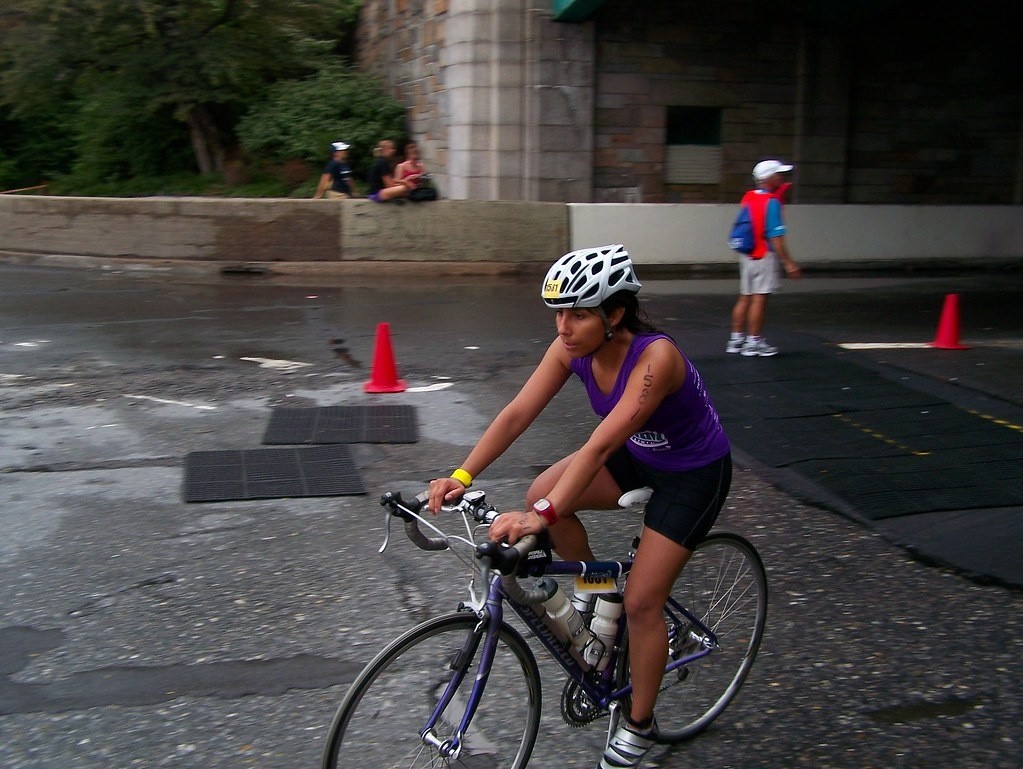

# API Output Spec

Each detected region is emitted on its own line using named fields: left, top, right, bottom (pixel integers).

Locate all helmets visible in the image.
left=541, top=244, right=642, bottom=309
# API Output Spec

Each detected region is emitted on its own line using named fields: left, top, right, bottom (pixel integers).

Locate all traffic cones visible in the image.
left=930, top=292, right=972, bottom=350
left=363, top=321, right=409, bottom=394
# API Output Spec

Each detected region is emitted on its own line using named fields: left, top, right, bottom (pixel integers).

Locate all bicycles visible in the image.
left=315, top=479, right=768, bottom=768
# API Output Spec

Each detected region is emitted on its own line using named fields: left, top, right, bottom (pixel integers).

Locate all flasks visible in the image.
left=534, top=578, right=590, bottom=650
left=584, top=591, right=625, bottom=671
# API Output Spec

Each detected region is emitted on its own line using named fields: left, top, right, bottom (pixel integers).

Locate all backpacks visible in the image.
left=728, top=207, right=753, bottom=255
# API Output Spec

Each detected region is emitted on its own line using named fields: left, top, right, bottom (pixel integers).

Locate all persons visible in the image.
left=726, top=160, right=800, bottom=357
left=313, top=142, right=359, bottom=199
left=368, top=139, right=428, bottom=201
left=430, top=245, right=734, bottom=769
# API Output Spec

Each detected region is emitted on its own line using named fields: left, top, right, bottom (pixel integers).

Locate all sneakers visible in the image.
left=741, top=339, right=778, bottom=356
left=726, top=338, right=746, bottom=353
left=570, top=595, right=591, bottom=621
left=597, top=719, right=658, bottom=769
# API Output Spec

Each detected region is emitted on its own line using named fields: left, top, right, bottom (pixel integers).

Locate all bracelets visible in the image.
left=450, top=469, right=472, bottom=488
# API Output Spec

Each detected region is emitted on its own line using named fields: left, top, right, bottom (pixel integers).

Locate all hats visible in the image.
left=329, top=142, right=351, bottom=152
left=752, top=160, right=794, bottom=180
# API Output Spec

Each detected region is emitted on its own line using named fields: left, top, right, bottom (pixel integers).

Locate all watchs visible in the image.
left=533, top=498, right=558, bottom=526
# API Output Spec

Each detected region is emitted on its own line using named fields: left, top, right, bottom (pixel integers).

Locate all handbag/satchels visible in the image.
left=409, top=187, right=436, bottom=202
